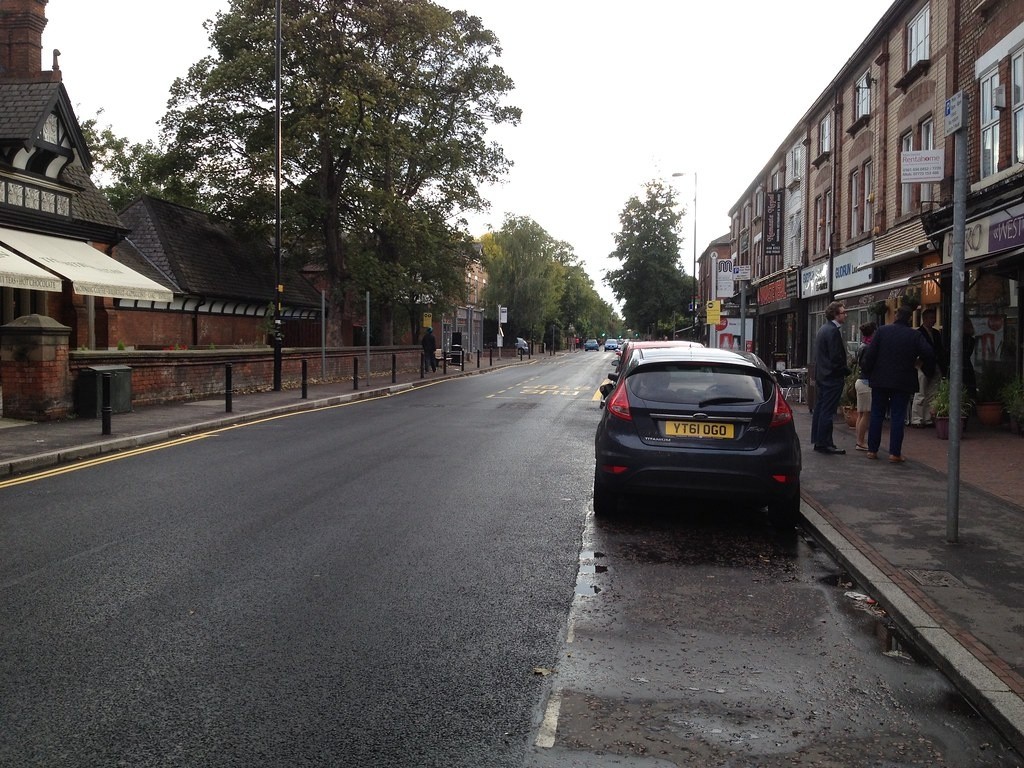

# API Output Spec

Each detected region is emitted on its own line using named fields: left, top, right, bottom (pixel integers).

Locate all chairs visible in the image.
left=770, top=370, right=805, bottom=404
left=640, top=372, right=675, bottom=402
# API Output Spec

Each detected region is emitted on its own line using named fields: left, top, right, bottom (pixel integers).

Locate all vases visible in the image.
left=976, top=401, right=1004, bottom=426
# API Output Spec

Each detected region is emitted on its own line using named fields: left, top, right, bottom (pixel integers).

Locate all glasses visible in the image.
left=839, top=311, right=845, bottom=315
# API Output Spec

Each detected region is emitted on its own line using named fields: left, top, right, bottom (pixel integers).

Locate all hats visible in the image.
left=427, top=327, right=433, bottom=333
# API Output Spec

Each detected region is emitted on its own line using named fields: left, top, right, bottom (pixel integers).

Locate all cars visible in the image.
left=592, top=346, right=802, bottom=539
left=597, top=337, right=707, bottom=410
left=516, top=337, right=528, bottom=356
left=603, top=339, right=618, bottom=351
left=585, top=340, right=599, bottom=351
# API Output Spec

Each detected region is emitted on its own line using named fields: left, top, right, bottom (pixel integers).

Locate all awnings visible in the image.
left=833, top=243, right=1024, bottom=301
left=0, top=225, right=173, bottom=303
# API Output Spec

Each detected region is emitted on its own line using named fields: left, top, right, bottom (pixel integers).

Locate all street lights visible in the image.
left=673, top=171, right=698, bottom=342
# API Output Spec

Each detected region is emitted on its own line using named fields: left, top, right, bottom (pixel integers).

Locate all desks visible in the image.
left=785, top=368, right=809, bottom=405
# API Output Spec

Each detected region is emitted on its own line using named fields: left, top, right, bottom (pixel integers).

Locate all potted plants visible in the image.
left=929, top=377, right=975, bottom=440
left=843, top=366, right=861, bottom=429
left=999, top=372, right=1024, bottom=433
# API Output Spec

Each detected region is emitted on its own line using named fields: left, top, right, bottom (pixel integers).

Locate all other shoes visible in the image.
left=434, top=369, right=436, bottom=373
left=887, top=454, right=906, bottom=463
left=426, top=372, right=429, bottom=374
left=913, top=424, right=925, bottom=429
left=866, top=452, right=876, bottom=460
left=926, top=424, right=935, bottom=428
left=856, top=445, right=869, bottom=451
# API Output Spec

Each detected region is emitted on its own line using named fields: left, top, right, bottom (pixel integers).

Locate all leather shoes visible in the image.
left=815, top=445, right=846, bottom=454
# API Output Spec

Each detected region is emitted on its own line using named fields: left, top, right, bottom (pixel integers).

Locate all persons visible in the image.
left=810, top=302, right=852, bottom=454
left=911, top=308, right=946, bottom=428
left=575, top=336, right=584, bottom=349
left=863, top=304, right=933, bottom=462
left=963, top=318, right=977, bottom=433
left=421, top=326, right=438, bottom=374
left=854, top=320, right=877, bottom=451
